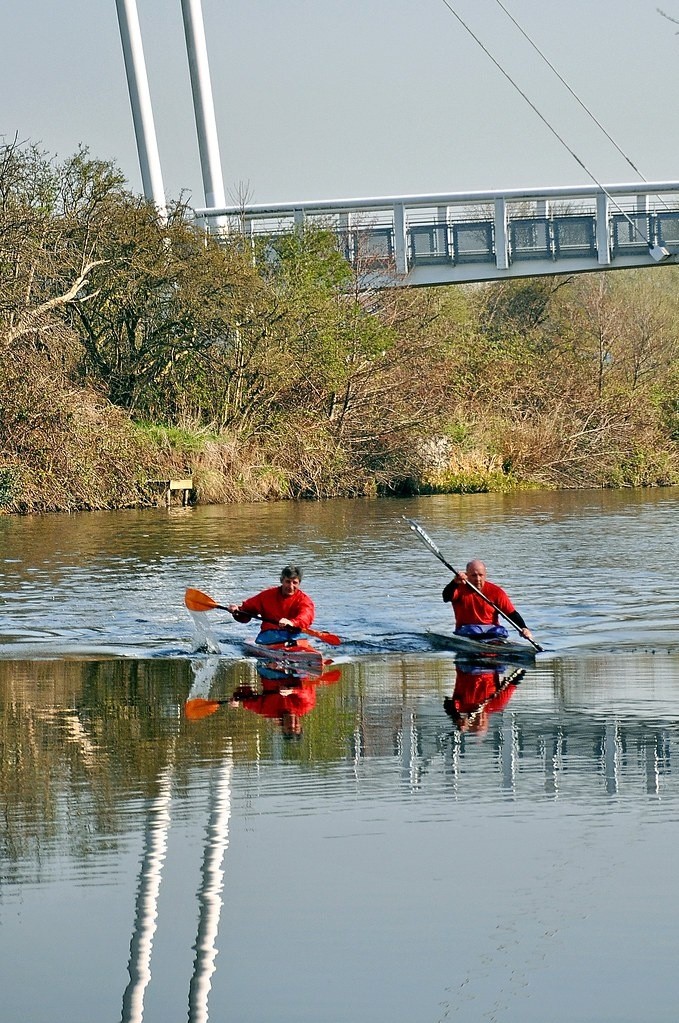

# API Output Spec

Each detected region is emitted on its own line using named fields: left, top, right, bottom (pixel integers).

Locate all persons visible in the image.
left=229, top=565, right=315, bottom=643
left=230, top=660, right=316, bottom=742
left=442, top=561, right=532, bottom=639
left=442, top=661, right=525, bottom=737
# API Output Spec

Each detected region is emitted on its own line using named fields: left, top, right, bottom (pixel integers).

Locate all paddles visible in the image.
left=185, top=668, right=341, bottom=721
left=405, top=517, right=547, bottom=654
left=185, top=587, right=342, bottom=646
left=451, top=667, right=522, bottom=739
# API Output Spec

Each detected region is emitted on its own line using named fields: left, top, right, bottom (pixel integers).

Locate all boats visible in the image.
left=419, top=624, right=537, bottom=665
left=243, top=635, right=324, bottom=673
